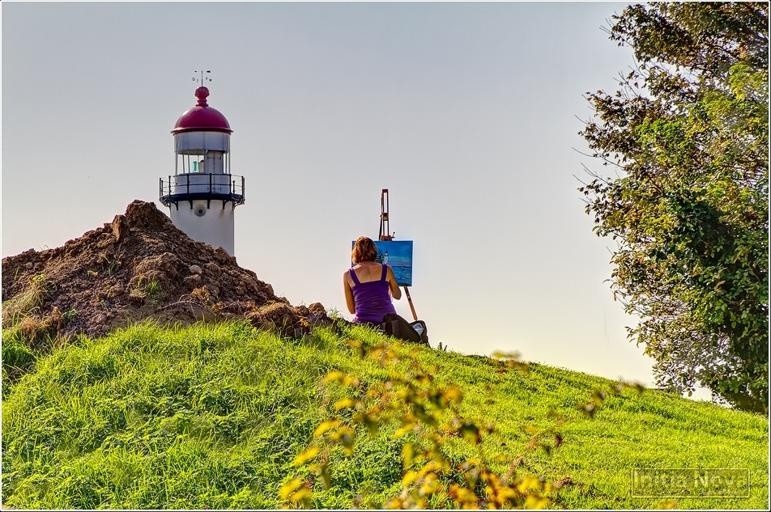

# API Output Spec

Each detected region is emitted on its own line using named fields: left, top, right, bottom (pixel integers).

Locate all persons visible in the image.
left=342, top=235, right=402, bottom=330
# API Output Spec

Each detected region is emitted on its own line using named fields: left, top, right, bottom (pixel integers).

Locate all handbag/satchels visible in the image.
left=380, top=313, right=428, bottom=344
